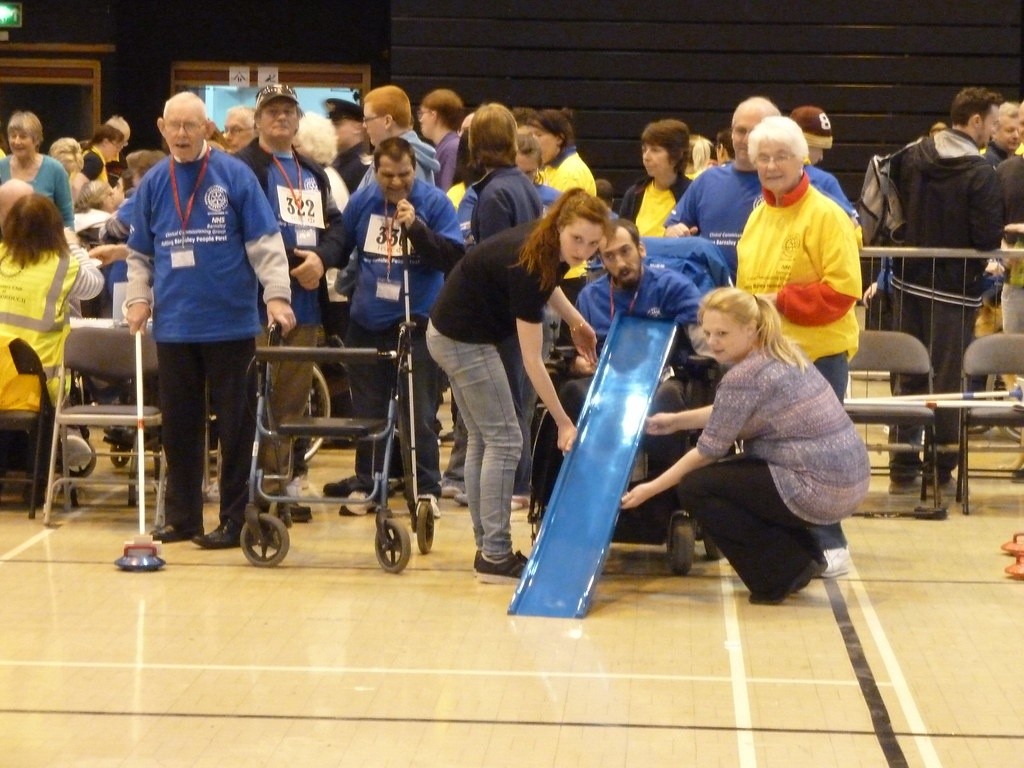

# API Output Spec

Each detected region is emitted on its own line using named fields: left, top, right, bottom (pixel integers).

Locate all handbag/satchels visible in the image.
left=857, top=137, right=923, bottom=246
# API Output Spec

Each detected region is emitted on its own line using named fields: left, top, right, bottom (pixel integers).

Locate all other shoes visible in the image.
left=454, top=494, right=469, bottom=505
left=924, top=477, right=970, bottom=497
left=288, top=503, right=312, bottom=522
left=749, top=557, right=828, bottom=605
left=512, top=497, right=530, bottom=511
left=888, top=474, right=923, bottom=494
left=323, top=476, right=374, bottom=496
left=441, top=479, right=464, bottom=498
left=670, top=517, right=694, bottom=575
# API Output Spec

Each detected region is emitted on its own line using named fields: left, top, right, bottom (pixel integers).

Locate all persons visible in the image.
left=1, top=84, right=1024, bottom=605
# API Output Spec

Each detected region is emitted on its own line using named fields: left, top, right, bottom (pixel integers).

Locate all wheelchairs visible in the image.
left=525, top=318, right=744, bottom=574
left=103, top=355, right=332, bottom=479
left=236, top=320, right=444, bottom=574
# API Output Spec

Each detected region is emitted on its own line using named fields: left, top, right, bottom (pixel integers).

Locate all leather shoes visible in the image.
left=193, top=519, right=242, bottom=548
left=149, top=524, right=205, bottom=543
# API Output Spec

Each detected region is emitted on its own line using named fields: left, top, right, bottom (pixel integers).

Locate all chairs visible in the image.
left=0, top=337, right=78, bottom=521
left=841, top=328, right=941, bottom=510
left=956, top=331, right=1024, bottom=517
left=41, top=325, right=168, bottom=532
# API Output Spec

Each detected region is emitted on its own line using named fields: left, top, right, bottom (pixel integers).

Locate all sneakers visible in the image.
left=339, top=491, right=381, bottom=516
left=416, top=494, right=441, bottom=518
left=811, top=546, right=851, bottom=577
left=473, top=550, right=529, bottom=585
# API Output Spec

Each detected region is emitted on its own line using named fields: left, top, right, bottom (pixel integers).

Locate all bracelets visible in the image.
left=570, top=321, right=587, bottom=331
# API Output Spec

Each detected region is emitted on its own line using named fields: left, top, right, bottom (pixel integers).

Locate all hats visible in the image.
left=325, top=98, right=363, bottom=120
left=789, top=106, right=833, bottom=149
left=254, top=84, right=300, bottom=114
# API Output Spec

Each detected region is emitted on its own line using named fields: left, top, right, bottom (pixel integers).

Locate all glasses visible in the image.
left=418, top=110, right=432, bottom=118
left=362, top=116, right=379, bottom=123
left=164, top=119, right=206, bottom=132
left=224, top=126, right=250, bottom=135
